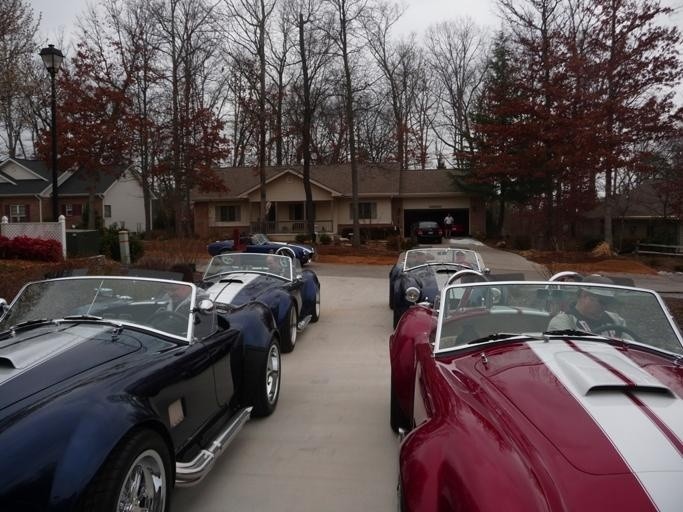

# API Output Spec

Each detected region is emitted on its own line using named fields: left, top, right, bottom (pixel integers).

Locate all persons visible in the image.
left=156, top=264, right=215, bottom=339
left=456, top=251, right=470, bottom=266
left=546, top=276, right=635, bottom=342
left=443, top=213, right=454, bottom=239
left=266, top=255, right=297, bottom=279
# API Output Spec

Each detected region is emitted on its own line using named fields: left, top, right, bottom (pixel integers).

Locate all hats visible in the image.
left=583, top=274, right=616, bottom=299
left=455, top=249, right=467, bottom=254
left=417, top=250, right=428, bottom=256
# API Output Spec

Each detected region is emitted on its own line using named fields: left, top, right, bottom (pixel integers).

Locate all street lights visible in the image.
left=40, top=44, right=64, bottom=221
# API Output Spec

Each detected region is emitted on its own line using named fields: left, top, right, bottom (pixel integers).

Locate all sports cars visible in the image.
left=176, top=253, right=321, bottom=353
left=208, top=234, right=316, bottom=267
left=390, top=248, right=510, bottom=330
left=0, top=277, right=281, bottom=512
left=387, top=267, right=682, bottom=511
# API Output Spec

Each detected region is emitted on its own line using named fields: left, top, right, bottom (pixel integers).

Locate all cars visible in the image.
left=411, top=221, right=444, bottom=244
